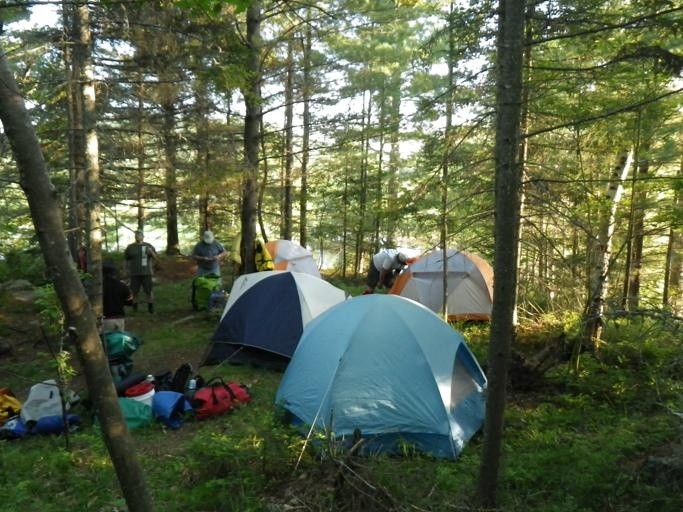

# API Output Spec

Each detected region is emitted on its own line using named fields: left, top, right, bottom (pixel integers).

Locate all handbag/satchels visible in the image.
left=192, top=378, right=251, bottom=419
left=0, top=386, right=23, bottom=421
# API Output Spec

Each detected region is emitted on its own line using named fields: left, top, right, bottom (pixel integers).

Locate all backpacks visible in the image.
left=100, top=327, right=144, bottom=385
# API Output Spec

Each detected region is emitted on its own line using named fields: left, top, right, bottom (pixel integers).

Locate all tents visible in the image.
left=256, top=238, right=321, bottom=281
left=274, top=293, right=487, bottom=473
left=200, top=267, right=352, bottom=374
left=387, top=249, right=495, bottom=324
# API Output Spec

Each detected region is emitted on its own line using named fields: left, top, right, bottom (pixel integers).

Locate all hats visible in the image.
left=202, top=230, right=213, bottom=244
left=398, top=251, right=408, bottom=264
left=102, top=262, right=120, bottom=278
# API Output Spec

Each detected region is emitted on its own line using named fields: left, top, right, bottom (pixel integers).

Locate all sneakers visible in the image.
left=148, top=303, right=154, bottom=313
left=133, top=302, right=138, bottom=311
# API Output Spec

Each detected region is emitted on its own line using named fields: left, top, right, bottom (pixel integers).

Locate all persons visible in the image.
left=361, top=249, right=416, bottom=295
left=100, top=262, right=133, bottom=333
left=190, top=230, right=227, bottom=275
left=122, top=228, right=161, bottom=316
